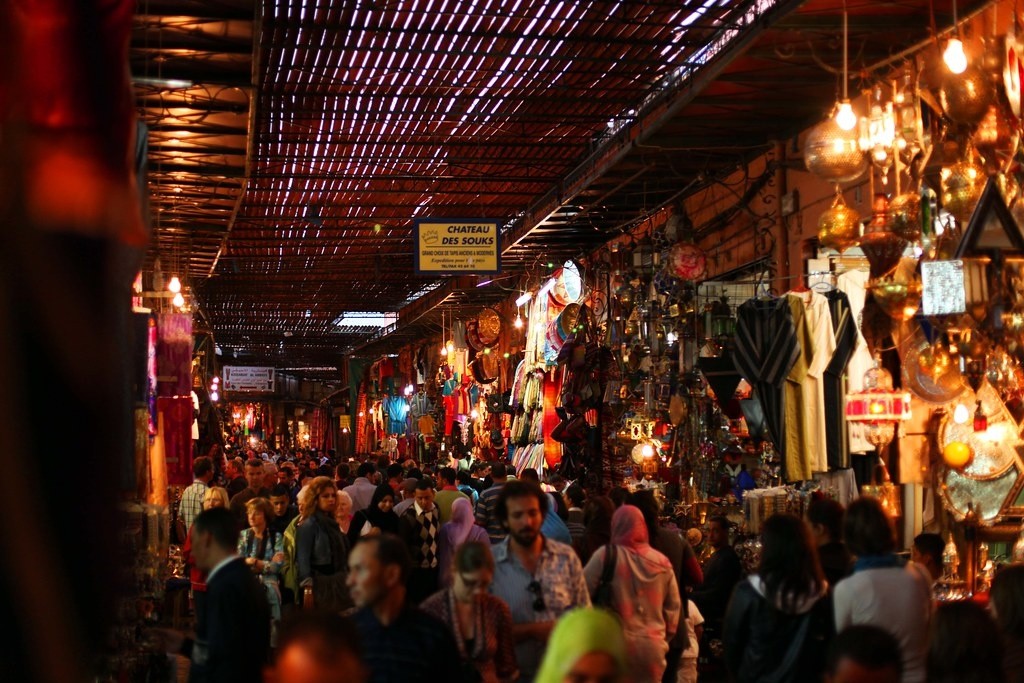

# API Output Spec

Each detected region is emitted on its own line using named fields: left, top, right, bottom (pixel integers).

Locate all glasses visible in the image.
left=300, top=463, right=306, bottom=466
left=459, top=573, right=494, bottom=589
left=527, top=581, right=546, bottom=613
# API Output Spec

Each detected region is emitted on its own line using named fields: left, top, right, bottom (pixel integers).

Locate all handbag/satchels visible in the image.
left=589, top=544, right=618, bottom=612
left=553, top=303, right=608, bottom=483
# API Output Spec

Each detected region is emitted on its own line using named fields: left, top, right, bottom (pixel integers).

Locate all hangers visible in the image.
left=751, top=256, right=870, bottom=301
left=413, top=385, right=428, bottom=395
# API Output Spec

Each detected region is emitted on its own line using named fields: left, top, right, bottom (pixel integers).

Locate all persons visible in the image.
left=178, top=446, right=1024, bottom=683
